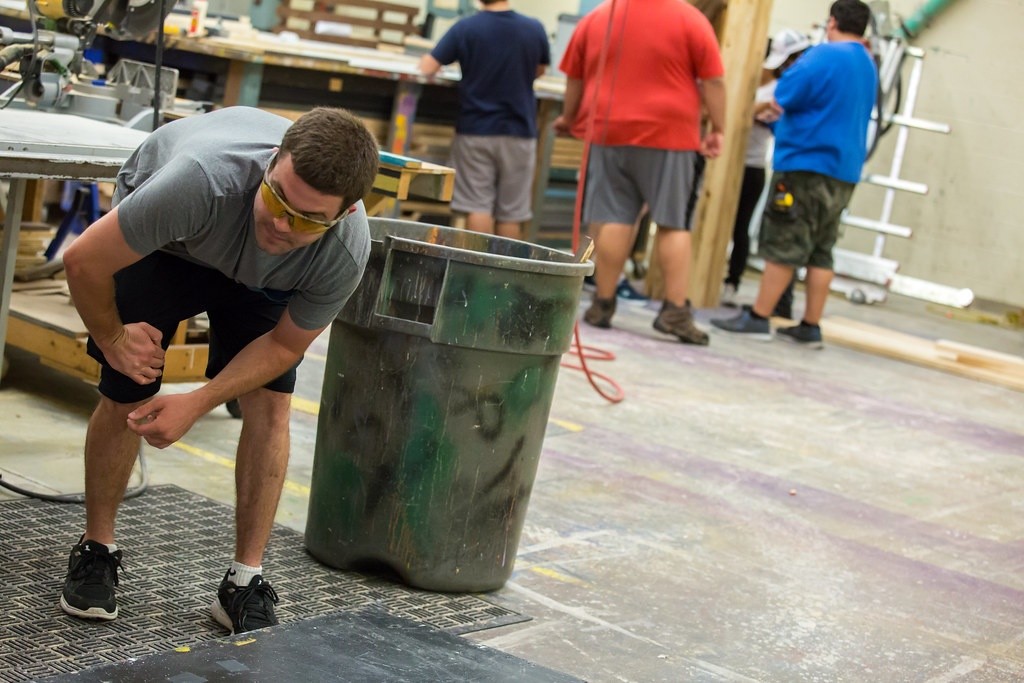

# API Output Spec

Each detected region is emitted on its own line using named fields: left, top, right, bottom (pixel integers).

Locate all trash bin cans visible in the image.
left=300, top=216, right=593, bottom=593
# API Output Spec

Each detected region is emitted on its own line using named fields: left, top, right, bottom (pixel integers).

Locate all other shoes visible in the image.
left=720, top=282, right=738, bottom=310
left=770, top=313, right=799, bottom=331
left=630, top=263, right=649, bottom=281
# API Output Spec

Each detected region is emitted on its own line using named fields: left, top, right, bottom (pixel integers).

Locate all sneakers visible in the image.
left=653, top=298, right=708, bottom=346
left=708, top=305, right=775, bottom=342
left=209, top=565, right=279, bottom=637
left=770, top=320, right=823, bottom=351
left=583, top=291, right=617, bottom=329
left=60, top=530, right=123, bottom=622
left=580, top=271, right=646, bottom=309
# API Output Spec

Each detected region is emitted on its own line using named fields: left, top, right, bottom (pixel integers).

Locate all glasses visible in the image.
left=261, top=150, right=349, bottom=235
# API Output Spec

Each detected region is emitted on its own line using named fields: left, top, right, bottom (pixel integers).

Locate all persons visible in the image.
left=58, top=104, right=381, bottom=637
left=711, top=1, right=882, bottom=350
left=721, top=40, right=780, bottom=301
left=759, top=28, right=814, bottom=321
left=418, top=0, right=550, bottom=242
left=550, top=0, right=726, bottom=345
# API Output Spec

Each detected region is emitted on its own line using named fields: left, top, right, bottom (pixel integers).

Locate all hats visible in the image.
left=762, top=28, right=811, bottom=69
